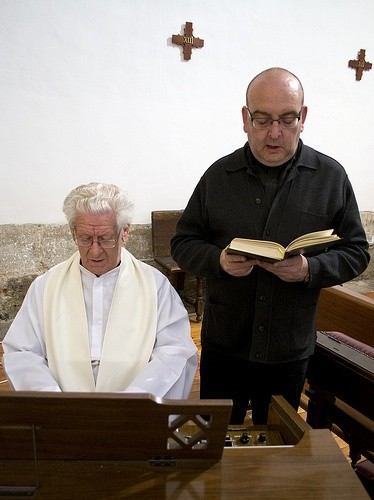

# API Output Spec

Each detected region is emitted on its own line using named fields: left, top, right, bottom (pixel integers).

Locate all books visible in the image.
left=224, top=228, right=344, bottom=264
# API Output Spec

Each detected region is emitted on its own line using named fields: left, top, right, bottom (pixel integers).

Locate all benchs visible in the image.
left=151, top=210, right=211, bottom=322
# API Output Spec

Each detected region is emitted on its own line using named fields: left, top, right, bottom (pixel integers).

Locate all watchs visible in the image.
left=304, top=272, right=311, bottom=283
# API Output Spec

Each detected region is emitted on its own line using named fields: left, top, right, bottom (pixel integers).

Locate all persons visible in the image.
left=169, top=67, right=371, bottom=425
left=2, top=181, right=198, bottom=424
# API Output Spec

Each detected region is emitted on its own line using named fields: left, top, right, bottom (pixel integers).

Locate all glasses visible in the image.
left=246, top=107, right=303, bottom=130
left=73, top=230, right=121, bottom=248
